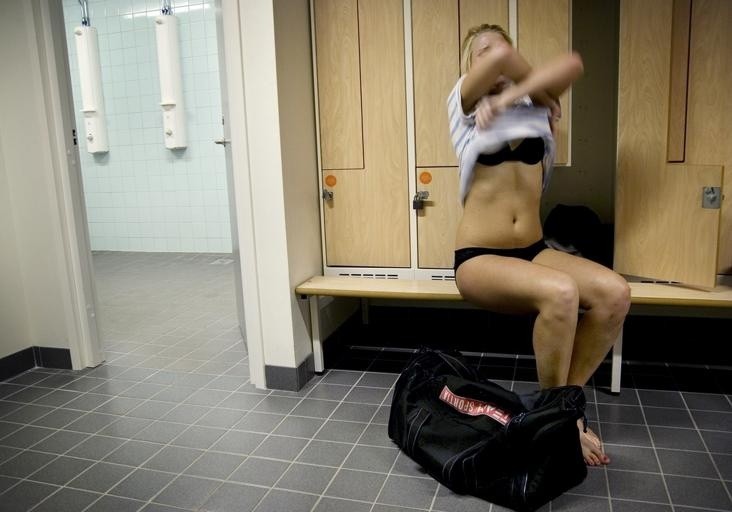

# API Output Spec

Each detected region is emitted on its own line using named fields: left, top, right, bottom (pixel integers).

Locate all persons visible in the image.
left=446, top=22, right=631, bottom=467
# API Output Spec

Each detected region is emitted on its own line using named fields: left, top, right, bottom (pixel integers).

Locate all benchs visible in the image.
left=296, top=276, right=730, bottom=395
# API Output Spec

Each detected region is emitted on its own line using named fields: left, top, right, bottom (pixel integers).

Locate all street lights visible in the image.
left=388, top=348, right=588, bottom=512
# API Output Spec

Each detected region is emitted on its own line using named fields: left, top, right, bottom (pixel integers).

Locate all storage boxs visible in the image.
left=296, top=276, right=730, bottom=395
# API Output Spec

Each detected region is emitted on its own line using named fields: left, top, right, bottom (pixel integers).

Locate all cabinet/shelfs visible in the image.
left=309, top=1, right=731, bottom=289
